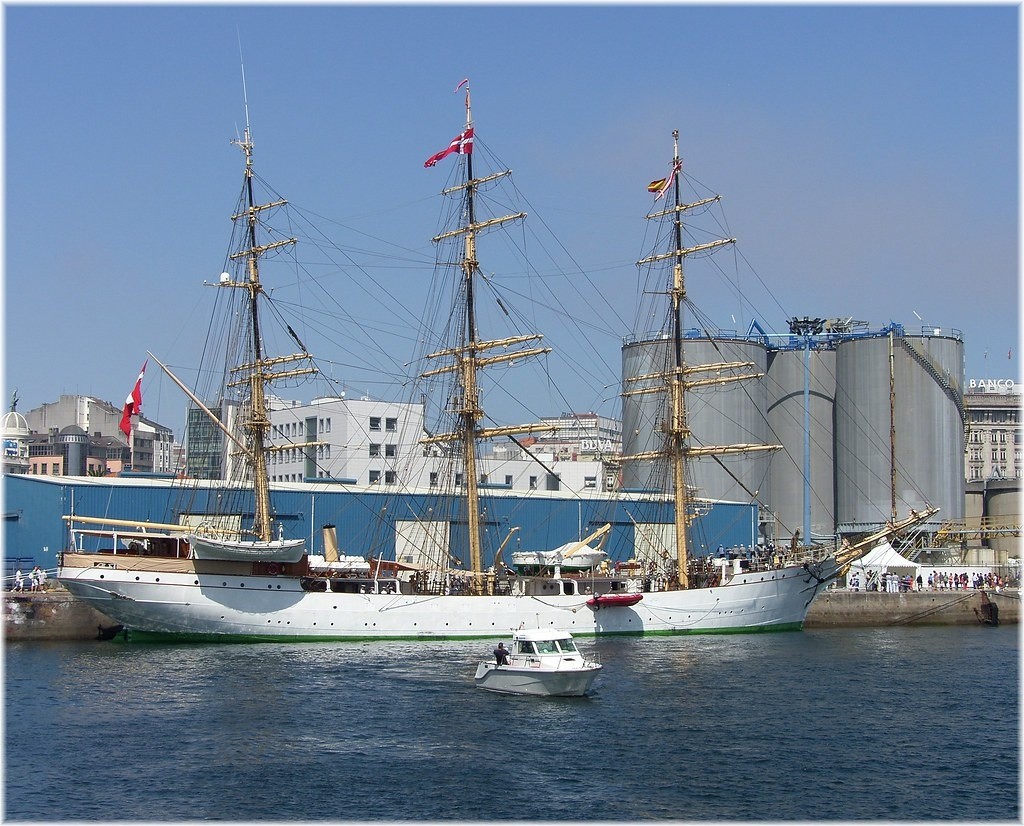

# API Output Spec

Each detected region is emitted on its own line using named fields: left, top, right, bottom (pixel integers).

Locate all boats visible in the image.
left=474, top=613, right=603, bottom=696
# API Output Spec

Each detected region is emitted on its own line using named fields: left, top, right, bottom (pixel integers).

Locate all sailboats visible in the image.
left=53, top=24, right=941, bottom=645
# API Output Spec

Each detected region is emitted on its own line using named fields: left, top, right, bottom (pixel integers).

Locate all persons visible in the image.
left=706, top=539, right=796, bottom=571
left=11, top=566, right=49, bottom=594
left=493, top=642, right=510, bottom=665
left=599, top=559, right=621, bottom=576
left=849, top=570, right=1021, bottom=592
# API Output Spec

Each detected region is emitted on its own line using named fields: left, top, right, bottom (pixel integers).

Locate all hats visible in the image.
left=719, top=544, right=722, bottom=547
left=748, top=545, right=751, bottom=548
left=740, top=544, right=743, bottom=547
left=785, top=544, right=788, bottom=547
left=734, top=544, right=737, bottom=547
left=755, top=545, right=757, bottom=547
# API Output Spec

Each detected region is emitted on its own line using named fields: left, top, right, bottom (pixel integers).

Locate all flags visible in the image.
left=425, top=128, right=474, bottom=167
left=119, top=359, right=147, bottom=445
